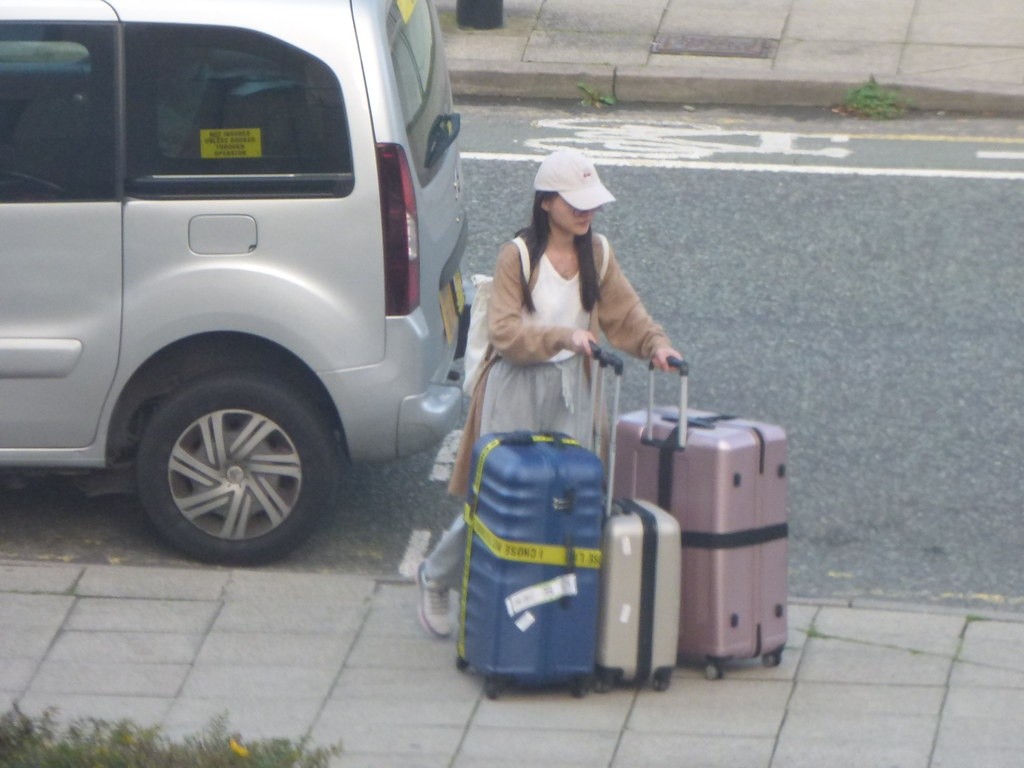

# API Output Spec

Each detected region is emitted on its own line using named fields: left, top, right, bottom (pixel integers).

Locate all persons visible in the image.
left=415, top=149, right=684, bottom=639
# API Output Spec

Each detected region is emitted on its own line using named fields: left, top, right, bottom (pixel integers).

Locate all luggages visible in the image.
left=455, top=339, right=603, bottom=702
left=594, top=351, right=682, bottom=692
left=616, top=351, right=789, bottom=692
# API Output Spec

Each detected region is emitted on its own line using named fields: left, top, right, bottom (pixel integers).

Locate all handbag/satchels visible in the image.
left=462, top=236, right=531, bottom=399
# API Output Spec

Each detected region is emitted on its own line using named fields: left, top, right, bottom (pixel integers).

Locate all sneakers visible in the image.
left=415, top=559, right=456, bottom=638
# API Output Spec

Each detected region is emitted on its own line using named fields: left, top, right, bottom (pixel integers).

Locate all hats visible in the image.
left=534, top=149, right=616, bottom=211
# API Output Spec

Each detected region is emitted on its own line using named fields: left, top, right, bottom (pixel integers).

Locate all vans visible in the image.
left=0, top=0, right=470, bottom=565
